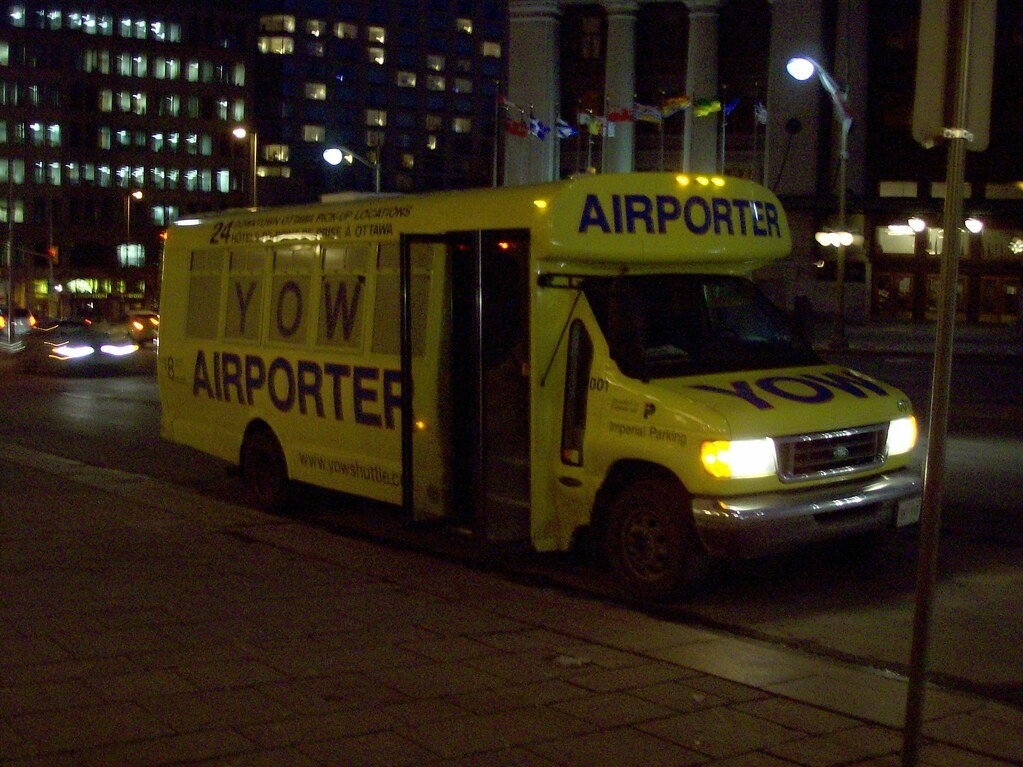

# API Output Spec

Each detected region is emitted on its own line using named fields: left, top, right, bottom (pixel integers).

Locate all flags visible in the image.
left=495, top=83, right=516, bottom=109
left=576, top=104, right=615, bottom=138
left=530, top=112, right=551, bottom=142
left=606, top=92, right=633, bottom=122
left=663, top=92, right=691, bottom=118
left=725, top=91, right=741, bottom=115
left=501, top=114, right=528, bottom=139
left=693, top=96, right=721, bottom=116
left=555, top=113, right=578, bottom=139
left=633, top=90, right=663, bottom=125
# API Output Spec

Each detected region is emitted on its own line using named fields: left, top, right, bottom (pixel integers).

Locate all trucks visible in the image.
left=155, top=172, right=922, bottom=601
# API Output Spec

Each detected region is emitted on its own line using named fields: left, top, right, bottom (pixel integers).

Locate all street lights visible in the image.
left=231, top=127, right=262, bottom=211
left=321, top=144, right=380, bottom=194
left=784, top=54, right=858, bottom=359
left=124, top=187, right=142, bottom=270
